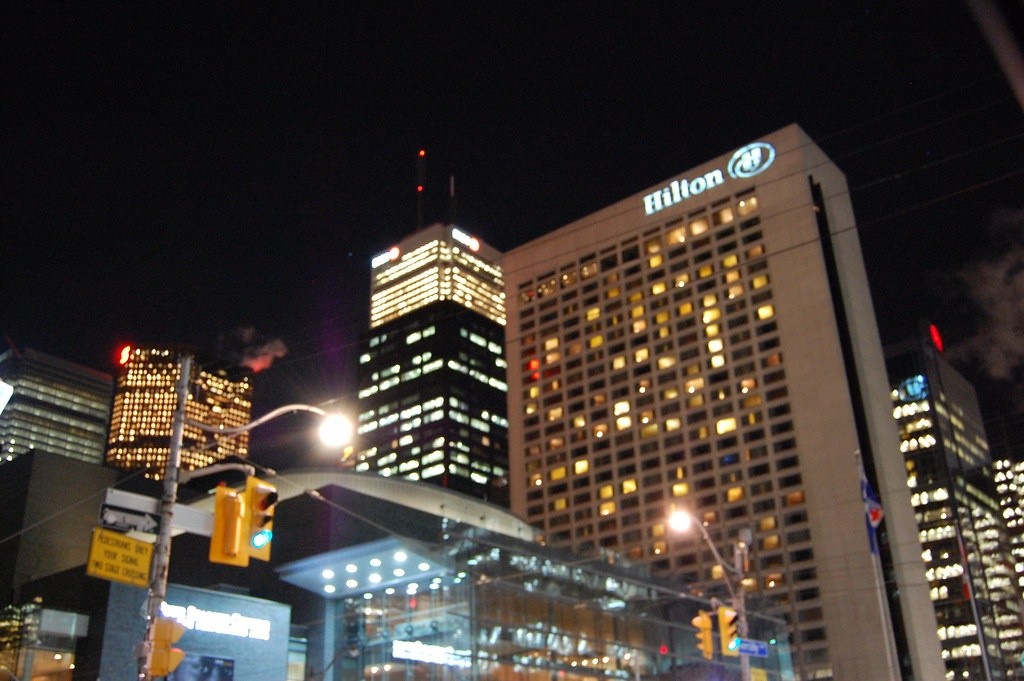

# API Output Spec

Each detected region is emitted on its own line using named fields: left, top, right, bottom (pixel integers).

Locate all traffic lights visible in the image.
left=717, top=605, right=741, bottom=656
left=251, top=478, right=279, bottom=562
left=691, top=609, right=713, bottom=658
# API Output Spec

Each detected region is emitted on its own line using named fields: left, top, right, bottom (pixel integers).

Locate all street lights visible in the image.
left=669, top=507, right=753, bottom=680
left=136, top=354, right=354, bottom=681
